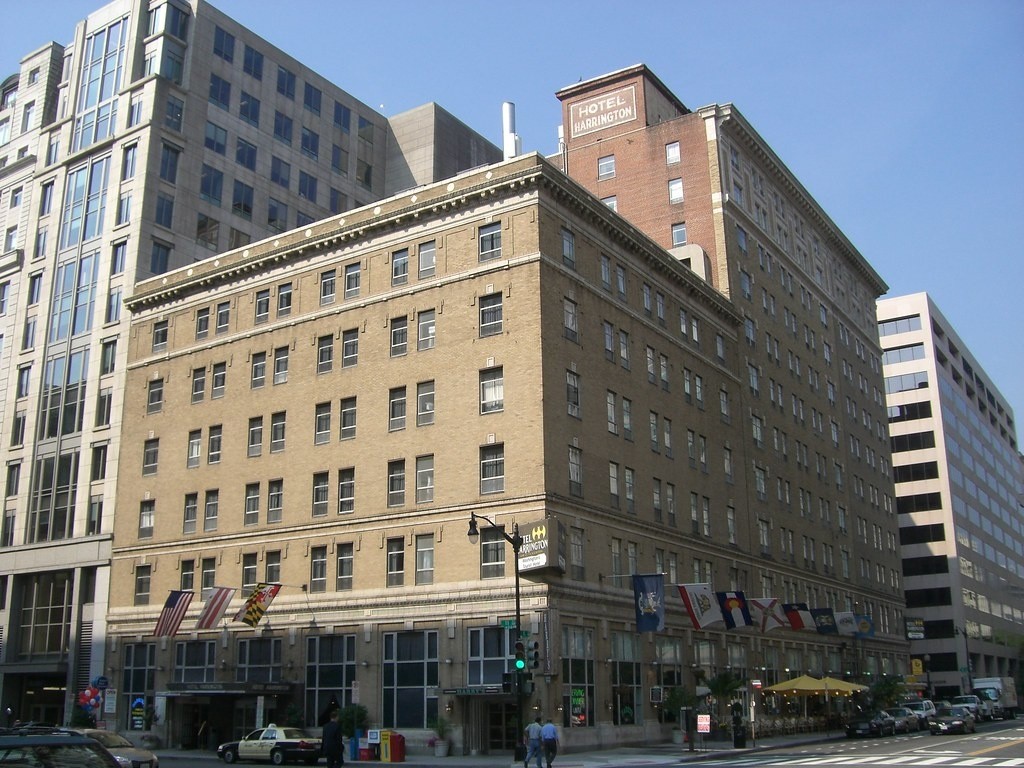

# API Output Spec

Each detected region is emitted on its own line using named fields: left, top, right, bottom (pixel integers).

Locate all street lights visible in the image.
left=467, top=511, right=527, bottom=762
left=954, top=627, right=972, bottom=696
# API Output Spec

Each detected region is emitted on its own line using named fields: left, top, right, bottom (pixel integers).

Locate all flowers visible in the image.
left=140, top=704, right=161, bottom=741
left=704, top=670, right=743, bottom=729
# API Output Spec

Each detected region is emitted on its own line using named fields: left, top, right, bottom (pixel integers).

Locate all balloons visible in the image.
left=78, top=686, right=103, bottom=712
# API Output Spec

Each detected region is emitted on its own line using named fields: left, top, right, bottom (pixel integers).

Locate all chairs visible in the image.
left=727, top=716, right=828, bottom=738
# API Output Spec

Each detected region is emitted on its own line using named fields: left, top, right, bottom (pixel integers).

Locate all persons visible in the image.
left=321, top=711, right=345, bottom=768
left=523, top=717, right=559, bottom=768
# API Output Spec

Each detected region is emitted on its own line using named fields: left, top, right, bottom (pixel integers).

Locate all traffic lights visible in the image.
left=517, top=673, right=534, bottom=698
left=515, top=641, right=525, bottom=670
left=528, top=642, right=539, bottom=671
left=501, top=670, right=515, bottom=694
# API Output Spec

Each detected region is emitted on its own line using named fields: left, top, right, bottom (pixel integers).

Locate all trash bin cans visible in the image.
left=733, top=726, right=746, bottom=748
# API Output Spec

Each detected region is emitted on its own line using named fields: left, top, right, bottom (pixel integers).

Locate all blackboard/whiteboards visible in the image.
left=650, top=687, right=663, bottom=702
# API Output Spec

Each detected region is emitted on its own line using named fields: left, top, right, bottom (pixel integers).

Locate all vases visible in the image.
left=713, top=729, right=730, bottom=742
left=143, top=740, right=159, bottom=749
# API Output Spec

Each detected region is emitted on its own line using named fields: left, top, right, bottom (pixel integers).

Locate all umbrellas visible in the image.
left=761, top=674, right=870, bottom=719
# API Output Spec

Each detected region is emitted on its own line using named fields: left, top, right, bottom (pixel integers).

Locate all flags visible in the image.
left=715, top=591, right=753, bottom=630
left=153, top=590, right=195, bottom=638
left=781, top=602, right=817, bottom=633
left=809, top=608, right=838, bottom=634
left=632, top=573, right=665, bottom=633
left=677, top=583, right=723, bottom=631
left=750, top=598, right=786, bottom=635
left=833, top=612, right=859, bottom=634
left=853, top=615, right=875, bottom=639
left=193, top=586, right=238, bottom=630
left=232, top=582, right=282, bottom=629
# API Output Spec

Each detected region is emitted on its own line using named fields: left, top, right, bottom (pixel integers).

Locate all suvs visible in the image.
left=901, top=700, right=936, bottom=731
left=975, top=692, right=995, bottom=722
left=951, top=695, right=983, bottom=722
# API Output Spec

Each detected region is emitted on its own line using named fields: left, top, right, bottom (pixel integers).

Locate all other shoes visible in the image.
left=524, top=760, right=528, bottom=768
left=547, top=762, right=552, bottom=768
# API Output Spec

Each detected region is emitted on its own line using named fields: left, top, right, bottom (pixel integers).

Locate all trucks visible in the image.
left=972, top=677, right=1017, bottom=719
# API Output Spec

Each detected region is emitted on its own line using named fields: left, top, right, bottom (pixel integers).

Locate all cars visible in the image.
left=883, top=707, right=920, bottom=733
left=217, top=725, right=324, bottom=767
left=932, top=700, right=951, bottom=710
left=928, top=708, right=977, bottom=735
left=0, top=725, right=159, bottom=768
left=844, top=711, right=895, bottom=737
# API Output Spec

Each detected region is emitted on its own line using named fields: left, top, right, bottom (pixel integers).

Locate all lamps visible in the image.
left=445, top=656, right=453, bottom=665
left=446, top=701, right=454, bottom=715
left=286, top=659, right=294, bottom=671
left=554, top=701, right=564, bottom=713
left=689, top=663, right=697, bottom=672
left=723, top=664, right=732, bottom=671
left=603, top=656, right=612, bottom=667
left=157, top=665, right=165, bottom=672
left=605, top=699, right=613, bottom=712
left=361, top=660, right=369, bottom=667
left=107, top=667, right=115, bottom=673
left=532, top=698, right=542, bottom=712
left=220, top=660, right=228, bottom=671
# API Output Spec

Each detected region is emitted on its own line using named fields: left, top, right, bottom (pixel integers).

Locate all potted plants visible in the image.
left=338, top=703, right=368, bottom=761
left=430, top=717, right=452, bottom=757
left=662, top=685, right=691, bottom=744
